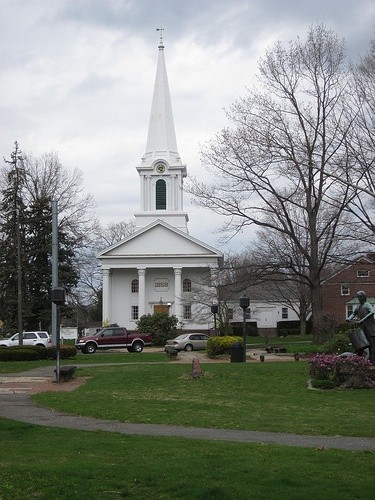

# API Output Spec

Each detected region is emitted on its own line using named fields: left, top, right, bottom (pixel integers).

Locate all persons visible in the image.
left=345, top=291, right=375, bottom=355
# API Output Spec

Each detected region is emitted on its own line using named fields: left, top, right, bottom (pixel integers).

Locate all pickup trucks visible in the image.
left=75, top=327, right=153, bottom=354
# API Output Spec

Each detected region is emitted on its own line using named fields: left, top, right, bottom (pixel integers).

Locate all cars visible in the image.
left=165, top=333, right=210, bottom=353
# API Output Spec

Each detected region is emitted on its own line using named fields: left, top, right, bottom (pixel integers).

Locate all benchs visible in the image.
left=266, top=347, right=286, bottom=353
left=54, top=366, right=76, bottom=382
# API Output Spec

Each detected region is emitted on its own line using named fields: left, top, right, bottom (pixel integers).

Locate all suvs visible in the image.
left=0, top=331, right=52, bottom=348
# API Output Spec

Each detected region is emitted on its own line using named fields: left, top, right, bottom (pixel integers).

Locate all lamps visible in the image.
left=167, top=303, right=172, bottom=309
